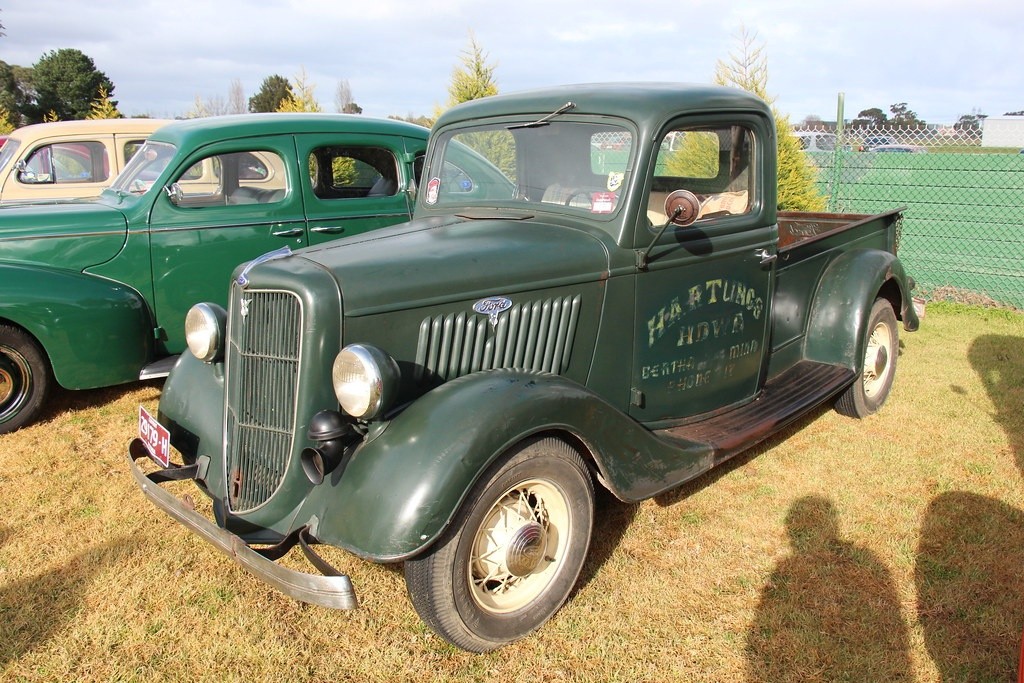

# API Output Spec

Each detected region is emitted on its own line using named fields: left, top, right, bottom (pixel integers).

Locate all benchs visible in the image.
left=542, top=183, right=747, bottom=226
left=228, top=186, right=286, bottom=205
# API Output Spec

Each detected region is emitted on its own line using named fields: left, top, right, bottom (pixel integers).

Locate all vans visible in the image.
left=790, top=131, right=853, bottom=153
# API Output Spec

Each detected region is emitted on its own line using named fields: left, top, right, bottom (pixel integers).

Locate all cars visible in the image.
left=859, top=136, right=900, bottom=153
left=872, top=143, right=929, bottom=153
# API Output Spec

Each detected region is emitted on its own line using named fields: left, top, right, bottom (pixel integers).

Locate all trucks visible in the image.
left=125, top=79, right=922, bottom=657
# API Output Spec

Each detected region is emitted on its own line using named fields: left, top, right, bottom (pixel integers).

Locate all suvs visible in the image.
left=0, top=112, right=520, bottom=436
left=0, top=117, right=316, bottom=203
